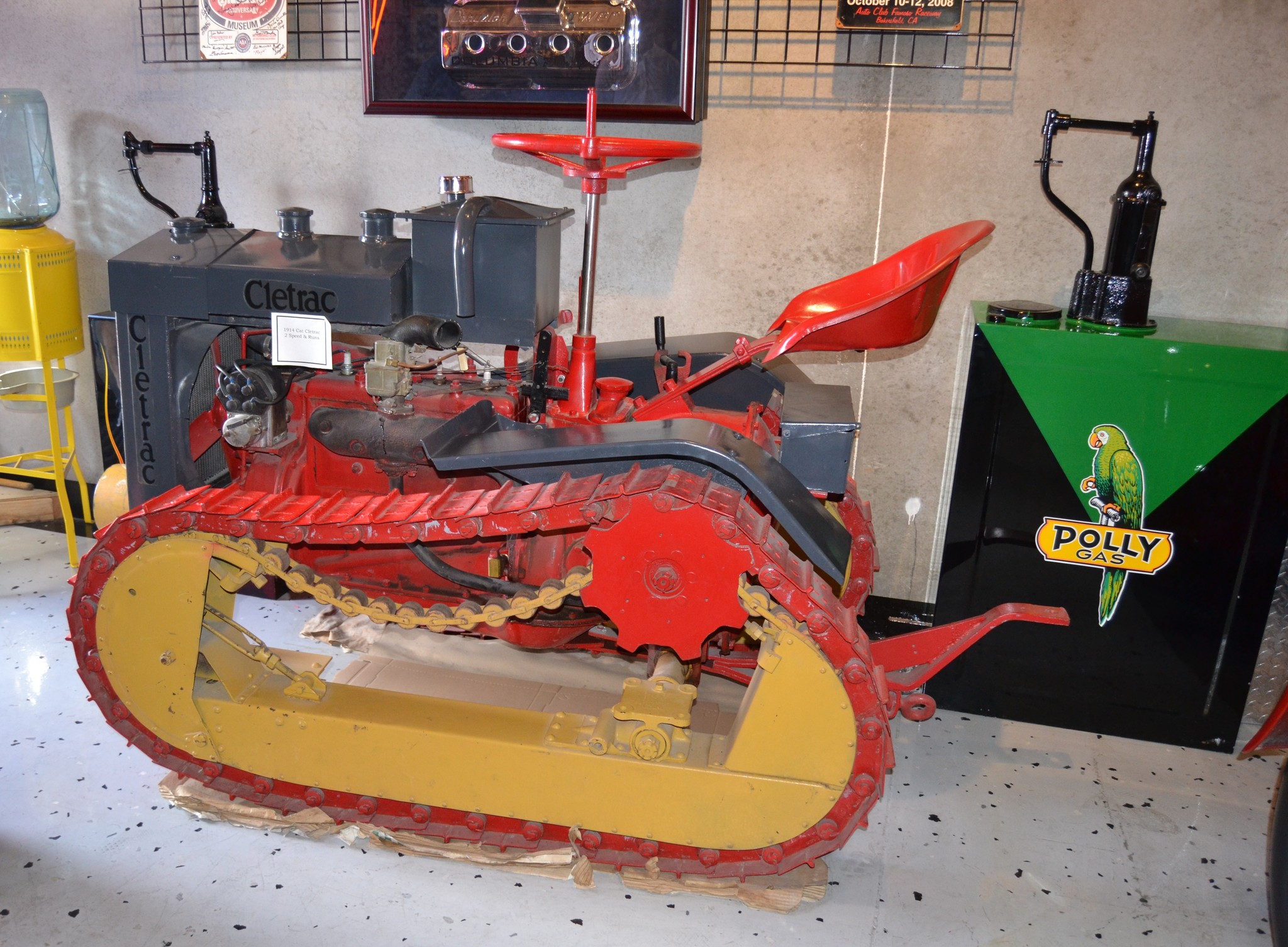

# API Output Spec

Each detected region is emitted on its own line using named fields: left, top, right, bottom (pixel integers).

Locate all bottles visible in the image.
left=0, top=88, right=60, bottom=232
